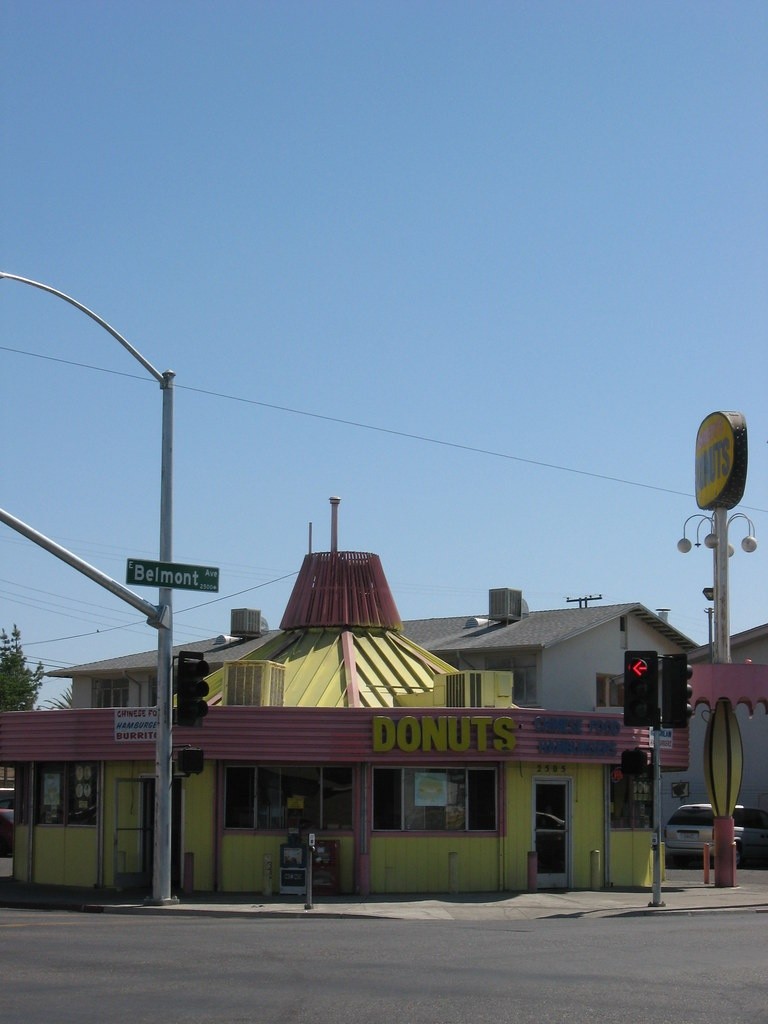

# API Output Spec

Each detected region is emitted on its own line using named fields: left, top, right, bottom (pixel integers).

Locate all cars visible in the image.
left=663, top=804, right=767, bottom=868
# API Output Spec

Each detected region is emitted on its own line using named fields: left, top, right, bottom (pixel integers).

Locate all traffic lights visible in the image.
left=624, top=651, right=659, bottom=736
left=178, top=651, right=209, bottom=725
left=663, top=652, right=694, bottom=728
